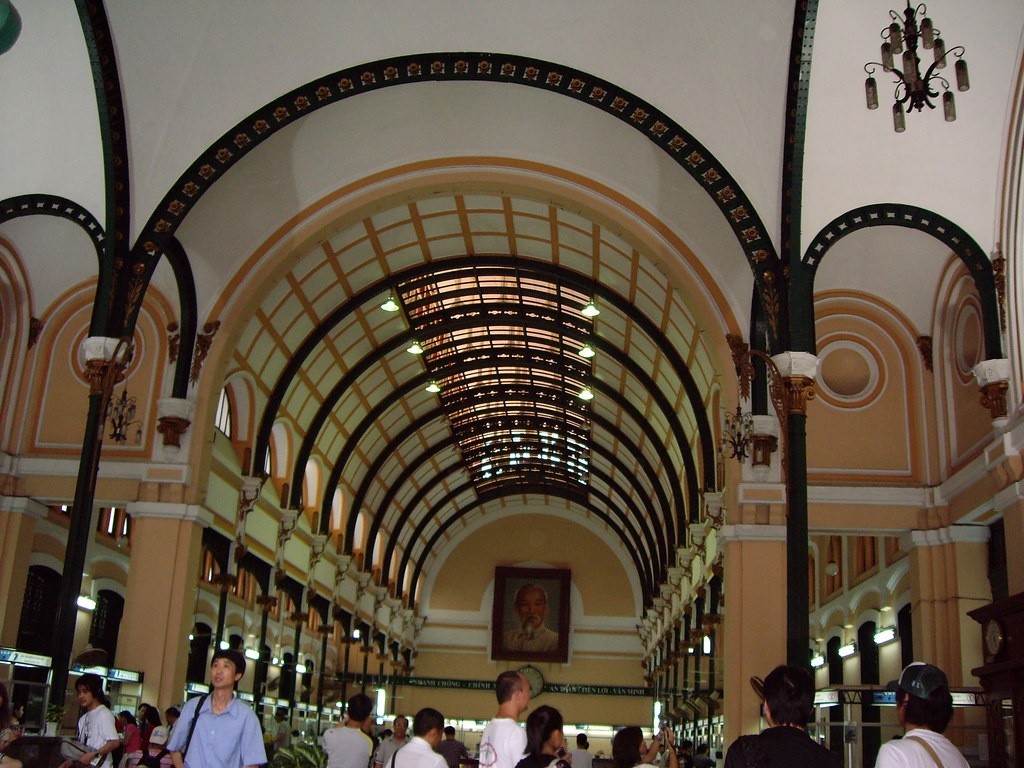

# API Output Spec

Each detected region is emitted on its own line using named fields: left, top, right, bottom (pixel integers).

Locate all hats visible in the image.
left=887, top=662, right=949, bottom=700
left=149, top=726, right=170, bottom=745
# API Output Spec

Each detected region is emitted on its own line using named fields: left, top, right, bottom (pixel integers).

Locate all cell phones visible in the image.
left=660, top=721, right=669, bottom=737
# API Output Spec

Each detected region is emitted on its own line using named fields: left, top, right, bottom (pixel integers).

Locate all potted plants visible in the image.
left=44, top=703, right=71, bottom=736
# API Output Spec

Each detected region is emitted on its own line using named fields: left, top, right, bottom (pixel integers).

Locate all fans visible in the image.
left=321, top=691, right=341, bottom=708
left=663, top=711, right=685, bottom=724
left=698, top=690, right=723, bottom=710
left=68, top=643, right=108, bottom=670
left=296, top=685, right=315, bottom=699
left=684, top=698, right=715, bottom=717
left=259, top=676, right=285, bottom=694
left=672, top=704, right=698, bottom=721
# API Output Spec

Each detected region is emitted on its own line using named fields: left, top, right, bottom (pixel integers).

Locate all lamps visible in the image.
left=715, top=402, right=753, bottom=464
left=407, top=340, right=424, bottom=354
left=864, top=0, right=970, bottom=133
left=213, top=639, right=230, bottom=650
left=826, top=536, right=838, bottom=576
left=244, top=647, right=260, bottom=660
left=380, top=288, right=400, bottom=313
left=76, top=596, right=100, bottom=612
left=578, top=387, right=594, bottom=400
left=581, top=298, right=600, bottom=317
left=425, top=378, right=441, bottom=393
left=811, top=655, right=825, bottom=667
left=296, top=664, right=307, bottom=673
left=105, top=360, right=143, bottom=445
left=874, top=624, right=897, bottom=644
left=578, top=344, right=596, bottom=358
left=838, top=641, right=857, bottom=658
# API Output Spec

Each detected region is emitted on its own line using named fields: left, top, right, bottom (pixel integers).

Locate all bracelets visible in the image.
left=95, top=750, right=101, bottom=758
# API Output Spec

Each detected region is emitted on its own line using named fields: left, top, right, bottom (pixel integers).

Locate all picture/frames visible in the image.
left=490, top=566, right=572, bottom=664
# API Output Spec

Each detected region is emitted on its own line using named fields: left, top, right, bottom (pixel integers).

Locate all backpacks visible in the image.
left=136, top=749, right=170, bottom=768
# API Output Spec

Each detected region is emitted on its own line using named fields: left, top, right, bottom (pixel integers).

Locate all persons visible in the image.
left=434, top=726, right=474, bottom=768
left=269, top=713, right=292, bottom=756
left=515, top=705, right=572, bottom=768
left=167, top=649, right=268, bottom=768
left=0, top=683, right=27, bottom=768
left=59, top=673, right=120, bottom=768
left=568, top=733, right=592, bottom=768
left=505, top=584, right=558, bottom=651
left=117, top=703, right=181, bottom=768
left=725, top=666, right=841, bottom=768
left=875, top=662, right=970, bottom=768
left=322, top=693, right=374, bottom=768
left=479, top=671, right=529, bottom=768
left=612, top=726, right=678, bottom=768
left=386, top=707, right=449, bottom=768
left=362, top=715, right=409, bottom=768
left=654, top=740, right=712, bottom=768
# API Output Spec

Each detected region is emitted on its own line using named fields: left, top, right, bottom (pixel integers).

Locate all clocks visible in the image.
left=516, top=664, right=544, bottom=700
